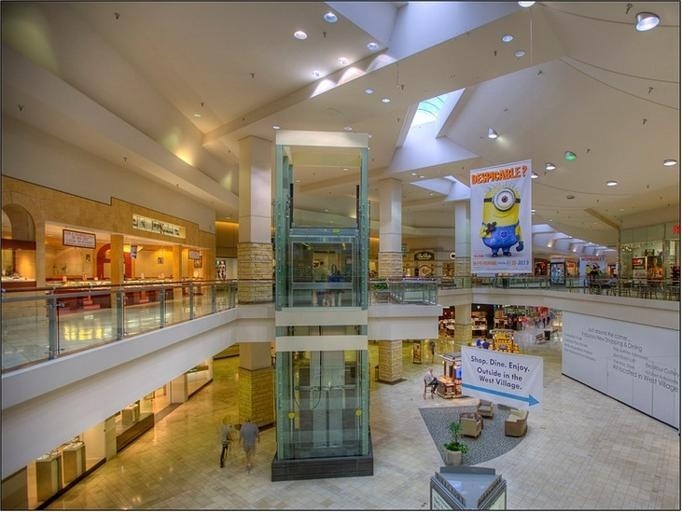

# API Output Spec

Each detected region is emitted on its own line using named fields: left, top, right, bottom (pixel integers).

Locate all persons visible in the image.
left=476, top=336, right=482, bottom=349
left=482, top=339, right=490, bottom=350
left=649, top=256, right=663, bottom=287
left=439, top=319, right=446, bottom=344
left=609, top=274, right=618, bottom=287
left=218, top=260, right=225, bottom=277
left=329, top=263, right=344, bottom=307
left=424, top=367, right=439, bottom=396
left=237, top=417, right=261, bottom=475
left=313, top=260, right=329, bottom=308
left=585, top=264, right=601, bottom=281
left=430, top=341, right=436, bottom=356
left=215, top=260, right=220, bottom=278
left=504, top=312, right=528, bottom=331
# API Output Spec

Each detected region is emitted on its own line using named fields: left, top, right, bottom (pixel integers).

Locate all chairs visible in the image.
left=459, top=396, right=529, bottom=438
left=425, top=379, right=434, bottom=399
left=589, top=264, right=681, bottom=300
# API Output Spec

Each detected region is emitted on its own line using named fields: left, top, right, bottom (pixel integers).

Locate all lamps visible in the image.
left=488, top=129, right=497, bottom=140
left=635, top=12, right=660, bottom=33
left=530, top=152, right=574, bottom=180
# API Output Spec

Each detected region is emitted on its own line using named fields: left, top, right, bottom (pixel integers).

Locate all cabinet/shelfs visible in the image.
left=37, top=385, right=170, bottom=496
left=428, top=466, right=507, bottom=510
left=3, top=274, right=201, bottom=312
left=444, top=307, right=551, bottom=340
left=438, top=350, right=468, bottom=399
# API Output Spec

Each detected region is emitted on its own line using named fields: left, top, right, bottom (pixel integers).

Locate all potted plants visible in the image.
left=445, top=419, right=468, bottom=467
